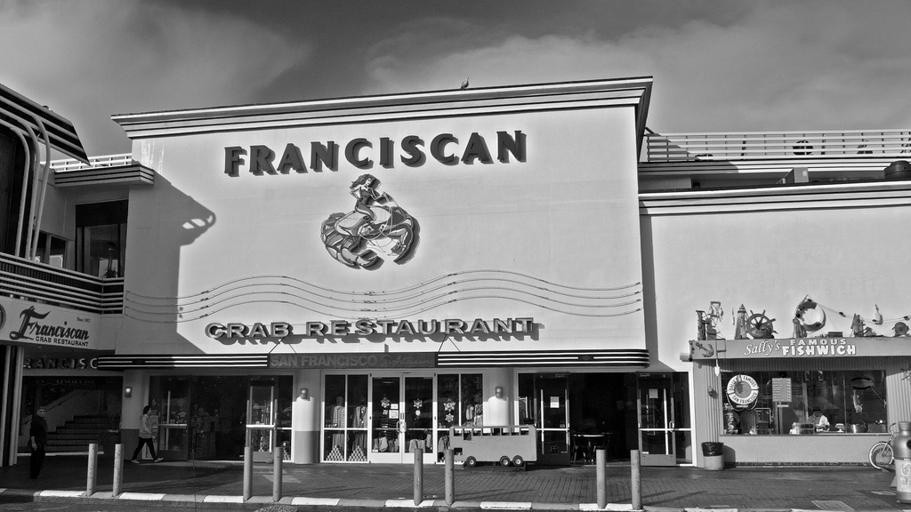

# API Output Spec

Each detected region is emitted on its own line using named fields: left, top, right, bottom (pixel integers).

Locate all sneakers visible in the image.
left=154, top=457, right=164, bottom=463
left=130, top=459, right=140, bottom=463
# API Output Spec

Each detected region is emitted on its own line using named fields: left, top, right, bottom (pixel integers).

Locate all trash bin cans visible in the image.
left=703, top=442, right=724, bottom=471
left=893, top=421, right=911, bottom=504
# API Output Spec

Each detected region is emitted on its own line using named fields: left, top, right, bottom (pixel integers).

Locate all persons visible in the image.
left=28, top=404, right=49, bottom=481
left=808, top=407, right=831, bottom=433
left=129, top=405, right=165, bottom=464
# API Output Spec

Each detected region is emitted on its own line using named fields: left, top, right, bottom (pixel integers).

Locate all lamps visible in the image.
left=125, top=386, right=132, bottom=397
left=495, top=387, right=503, bottom=399
left=300, top=388, right=307, bottom=399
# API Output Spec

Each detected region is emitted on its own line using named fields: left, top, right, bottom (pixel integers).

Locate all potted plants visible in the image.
left=106, top=394, right=122, bottom=444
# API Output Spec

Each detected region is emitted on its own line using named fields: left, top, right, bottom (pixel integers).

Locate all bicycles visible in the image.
left=868, top=421, right=899, bottom=470
